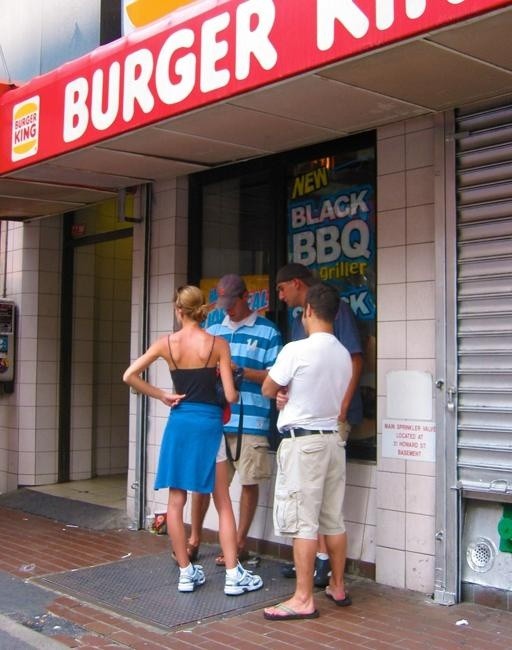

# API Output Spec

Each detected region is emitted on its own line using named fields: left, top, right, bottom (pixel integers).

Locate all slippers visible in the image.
left=264, top=603, right=319, bottom=620
left=325, top=587, right=352, bottom=606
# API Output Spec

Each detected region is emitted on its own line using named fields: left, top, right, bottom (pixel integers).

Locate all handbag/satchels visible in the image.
left=216, top=369, right=242, bottom=410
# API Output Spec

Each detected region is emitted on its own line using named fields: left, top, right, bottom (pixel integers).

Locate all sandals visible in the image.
left=215, top=546, right=248, bottom=566
left=171, top=538, right=197, bottom=562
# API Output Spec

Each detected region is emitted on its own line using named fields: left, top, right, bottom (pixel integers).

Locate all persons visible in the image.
left=261, top=283, right=354, bottom=620
left=275, top=262, right=364, bottom=587
left=170, top=273, right=284, bottom=561
left=123, top=286, right=263, bottom=595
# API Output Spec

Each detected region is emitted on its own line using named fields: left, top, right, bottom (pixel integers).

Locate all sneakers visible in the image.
left=279, top=557, right=331, bottom=586
left=224, top=561, right=263, bottom=595
left=177, top=563, right=205, bottom=592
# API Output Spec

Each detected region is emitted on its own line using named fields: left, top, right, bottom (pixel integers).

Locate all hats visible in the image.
left=216, top=274, right=245, bottom=310
left=275, top=264, right=315, bottom=286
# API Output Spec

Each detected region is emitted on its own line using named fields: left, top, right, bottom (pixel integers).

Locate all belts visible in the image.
left=283, top=429, right=338, bottom=438
